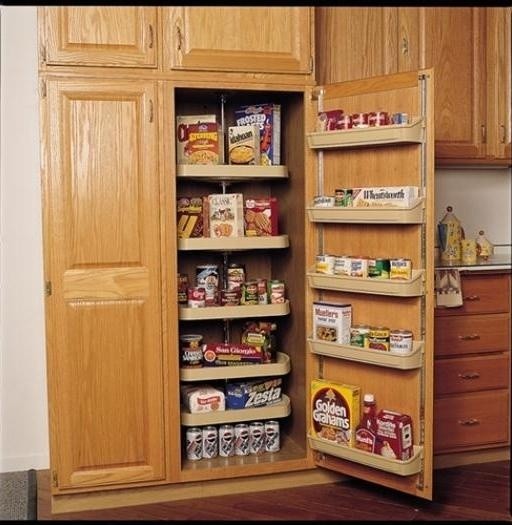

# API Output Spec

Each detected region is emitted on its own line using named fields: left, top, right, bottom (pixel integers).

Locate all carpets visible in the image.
left=1, top=468, right=39, bottom=521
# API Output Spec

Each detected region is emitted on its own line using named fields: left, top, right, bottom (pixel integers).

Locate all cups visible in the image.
left=461, top=240, right=478, bottom=265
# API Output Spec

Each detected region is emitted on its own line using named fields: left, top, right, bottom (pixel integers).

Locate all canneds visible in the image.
left=234, top=424, right=249, bottom=456
left=187, top=279, right=285, bottom=309
left=351, top=325, right=413, bottom=353
left=186, top=428, right=202, bottom=460
left=334, top=188, right=353, bottom=208
left=202, top=426, right=218, bottom=458
left=265, top=421, right=281, bottom=452
left=315, top=254, right=412, bottom=280
left=249, top=422, right=265, bottom=454
left=219, top=424, right=234, bottom=457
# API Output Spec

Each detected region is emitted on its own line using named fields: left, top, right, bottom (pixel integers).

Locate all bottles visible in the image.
left=476, top=230, right=495, bottom=264
left=354, top=393, right=376, bottom=455
left=437, top=205, right=461, bottom=264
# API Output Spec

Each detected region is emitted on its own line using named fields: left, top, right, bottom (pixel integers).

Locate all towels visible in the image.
left=432, top=270, right=463, bottom=308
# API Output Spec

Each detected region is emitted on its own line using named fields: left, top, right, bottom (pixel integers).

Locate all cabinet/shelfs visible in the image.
left=434, top=265, right=512, bottom=471
left=37, top=4, right=316, bottom=79
left=315, top=4, right=512, bottom=168
left=41, top=69, right=440, bottom=516
